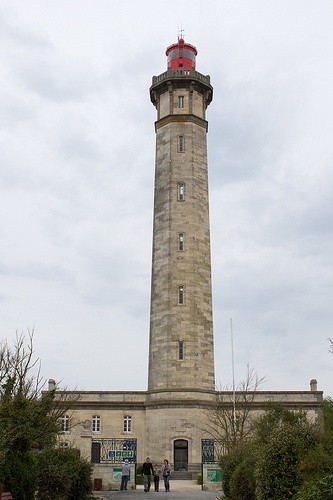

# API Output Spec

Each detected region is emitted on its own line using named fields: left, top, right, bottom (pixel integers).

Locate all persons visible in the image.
left=161, top=460, right=172, bottom=492
left=153, top=464, right=161, bottom=492
left=120, top=459, right=130, bottom=491
left=142, top=457, right=155, bottom=492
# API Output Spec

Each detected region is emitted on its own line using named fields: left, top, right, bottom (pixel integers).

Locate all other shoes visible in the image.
left=144, top=490, right=147, bottom=492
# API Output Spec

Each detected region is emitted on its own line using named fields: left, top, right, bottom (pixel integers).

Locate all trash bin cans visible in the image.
left=94, top=478, right=102, bottom=491
